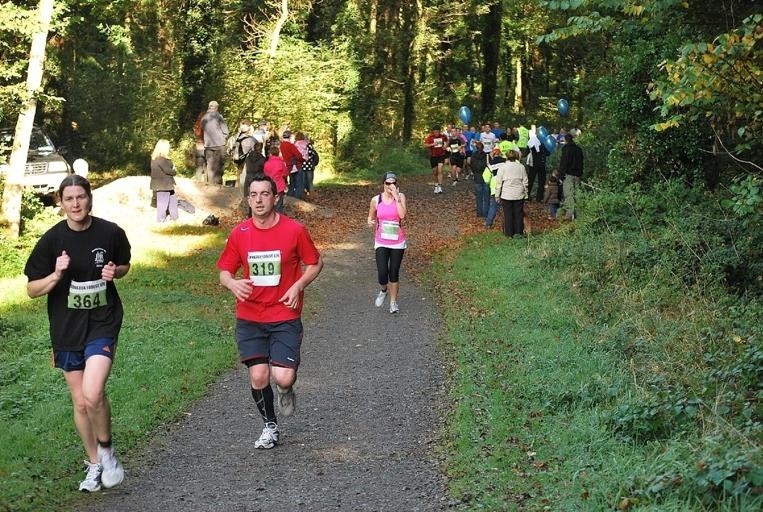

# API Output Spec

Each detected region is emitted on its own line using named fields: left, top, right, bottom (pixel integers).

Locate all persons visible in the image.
left=424, top=122, right=581, bottom=239
left=366, top=171, right=406, bottom=313
left=216, top=174, right=323, bottom=450
left=192, top=101, right=230, bottom=183
left=24, top=175, right=131, bottom=492
left=149, top=139, right=178, bottom=221
left=229, top=117, right=319, bottom=199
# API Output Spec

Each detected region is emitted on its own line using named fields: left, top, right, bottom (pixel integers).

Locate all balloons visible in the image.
left=459, top=104, right=470, bottom=124
left=557, top=97, right=570, bottom=120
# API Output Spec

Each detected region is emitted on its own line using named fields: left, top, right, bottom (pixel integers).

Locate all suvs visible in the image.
left=0, top=127, right=72, bottom=207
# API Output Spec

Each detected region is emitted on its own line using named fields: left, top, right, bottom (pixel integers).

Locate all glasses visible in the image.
left=385, top=182, right=395, bottom=185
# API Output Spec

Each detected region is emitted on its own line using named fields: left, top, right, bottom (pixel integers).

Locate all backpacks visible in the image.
left=233, top=137, right=248, bottom=163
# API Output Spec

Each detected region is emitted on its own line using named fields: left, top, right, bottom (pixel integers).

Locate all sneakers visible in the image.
left=78, top=460, right=103, bottom=493
left=276, top=385, right=296, bottom=418
left=253, top=422, right=279, bottom=450
left=375, top=290, right=387, bottom=308
left=425, top=170, right=473, bottom=194
left=97, top=444, right=123, bottom=489
left=389, top=304, right=399, bottom=314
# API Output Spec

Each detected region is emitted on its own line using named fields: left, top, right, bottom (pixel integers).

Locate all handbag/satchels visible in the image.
left=311, top=150, right=318, bottom=165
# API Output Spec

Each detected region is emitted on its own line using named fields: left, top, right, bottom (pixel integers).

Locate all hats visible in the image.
left=284, top=131, right=290, bottom=137
left=382, top=171, right=396, bottom=184
left=208, top=100, right=219, bottom=107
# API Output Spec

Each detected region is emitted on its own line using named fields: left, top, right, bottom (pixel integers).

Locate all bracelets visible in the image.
left=397, top=199, right=401, bottom=203
